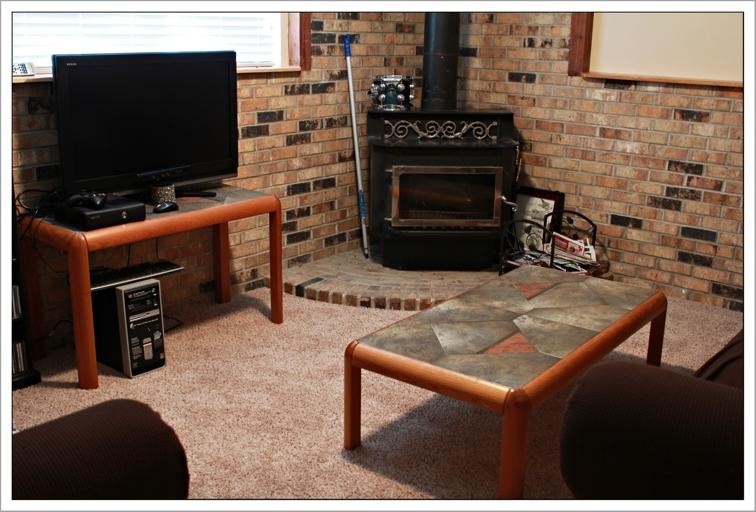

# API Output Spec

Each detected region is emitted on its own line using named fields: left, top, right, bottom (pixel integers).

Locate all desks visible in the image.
left=18, top=185, right=283, bottom=389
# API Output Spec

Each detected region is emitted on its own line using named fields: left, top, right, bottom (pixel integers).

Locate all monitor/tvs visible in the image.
left=52, top=50, right=238, bottom=197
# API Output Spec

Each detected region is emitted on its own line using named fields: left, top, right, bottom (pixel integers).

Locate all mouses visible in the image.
left=155, top=203, right=179, bottom=212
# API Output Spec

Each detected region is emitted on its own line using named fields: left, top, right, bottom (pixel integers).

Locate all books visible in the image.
left=507, top=232, right=598, bottom=275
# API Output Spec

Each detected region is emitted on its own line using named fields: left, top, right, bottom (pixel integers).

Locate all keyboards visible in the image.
left=90, top=259, right=184, bottom=290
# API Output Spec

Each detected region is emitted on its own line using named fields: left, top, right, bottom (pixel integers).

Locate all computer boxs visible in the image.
left=92, top=279, right=166, bottom=378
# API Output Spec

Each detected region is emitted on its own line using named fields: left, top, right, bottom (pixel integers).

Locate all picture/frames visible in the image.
left=510, top=184, right=564, bottom=250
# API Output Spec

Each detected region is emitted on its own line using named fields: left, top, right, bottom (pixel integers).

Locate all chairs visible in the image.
left=12, top=400, right=189, bottom=499
left=560, top=329, right=743, bottom=500
